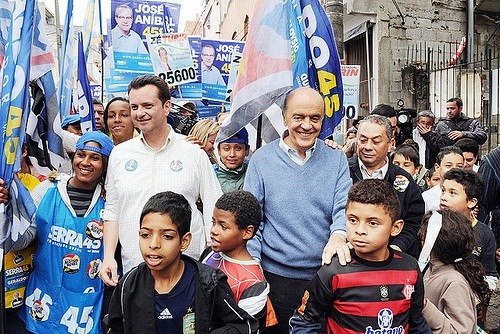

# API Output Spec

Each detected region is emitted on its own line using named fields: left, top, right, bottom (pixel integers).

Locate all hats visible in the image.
left=75, top=130, right=114, bottom=157
left=221, top=121, right=249, bottom=144
left=61, top=115, right=81, bottom=130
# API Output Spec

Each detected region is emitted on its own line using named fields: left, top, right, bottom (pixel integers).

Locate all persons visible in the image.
left=439, top=168, right=497, bottom=321
left=199, top=190, right=269, bottom=334
left=100, top=75, right=224, bottom=286
left=324, top=114, right=425, bottom=261
left=212, top=121, right=251, bottom=193
left=111, top=4, right=148, bottom=54
left=201, top=45, right=226, bottom=86
left=16, top=97, right=487, bottom=176
left=108, top=191, right=260, bottom=334
left=155, top=47, right=174, bottom=72
left=242, top=86, right=354, bottom=334
left=476, top=146, right=500, bottom=280
left=0, top=131, right=122, bottom=334
left=416, top=209, right=492, bottom=334
left=496, top=248, right=500, bottom=263
left=288, top=179, right=432, bottom=334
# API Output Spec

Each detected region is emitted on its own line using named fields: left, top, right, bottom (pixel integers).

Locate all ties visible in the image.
left=167, top=63, right=172, bottom=70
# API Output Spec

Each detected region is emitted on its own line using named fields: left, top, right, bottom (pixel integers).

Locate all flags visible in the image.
left=214, top=1, right=293, bottom=153
